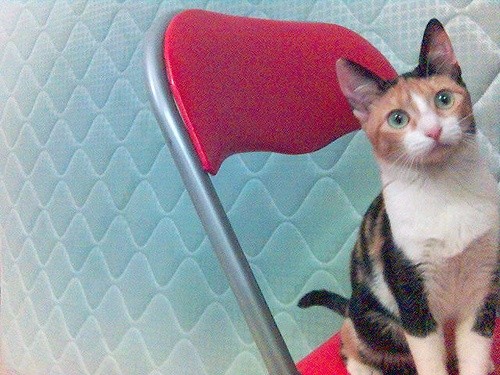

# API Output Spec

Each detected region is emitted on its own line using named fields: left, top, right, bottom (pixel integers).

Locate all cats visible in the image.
left=296, top=17, right=500, bottom=375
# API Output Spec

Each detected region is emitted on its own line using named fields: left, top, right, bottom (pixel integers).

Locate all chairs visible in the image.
left=144, top=8, right=499, bottom=374
